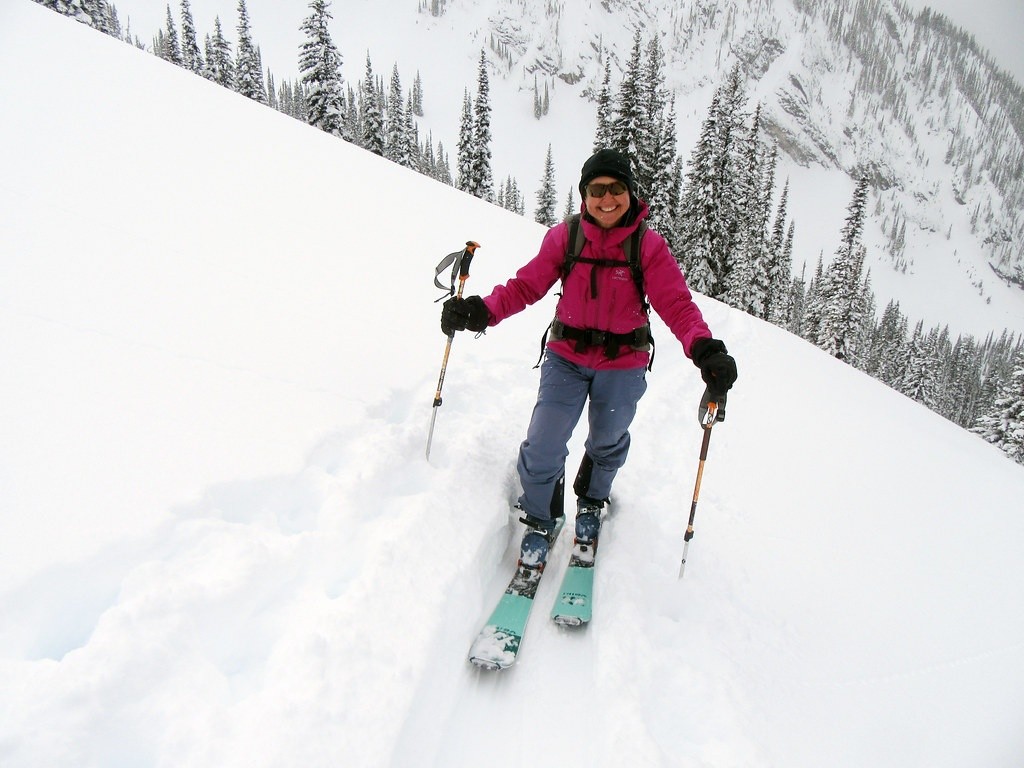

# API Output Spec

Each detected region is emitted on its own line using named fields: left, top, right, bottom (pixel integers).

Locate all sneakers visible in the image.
left=519, top=514, right=556, bottom=569
left=575, top=497, right=604, bottom=543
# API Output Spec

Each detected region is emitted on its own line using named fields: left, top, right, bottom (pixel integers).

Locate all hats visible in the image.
left=579, top=149, right=633, bottom=200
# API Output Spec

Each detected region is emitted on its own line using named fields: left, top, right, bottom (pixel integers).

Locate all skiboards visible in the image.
left=467, top=512, right=602, bottom=671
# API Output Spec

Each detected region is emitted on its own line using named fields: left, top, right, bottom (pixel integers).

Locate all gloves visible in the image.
left=692, top=338, right=737, bottom=396
left=441, top=296, right=488, bottom=339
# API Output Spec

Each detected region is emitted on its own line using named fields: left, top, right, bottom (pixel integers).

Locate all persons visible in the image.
left=436, top=150, right=739, bottom=571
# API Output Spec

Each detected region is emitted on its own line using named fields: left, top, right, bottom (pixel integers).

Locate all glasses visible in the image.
left=584, top=181, right=627, bottom=198
